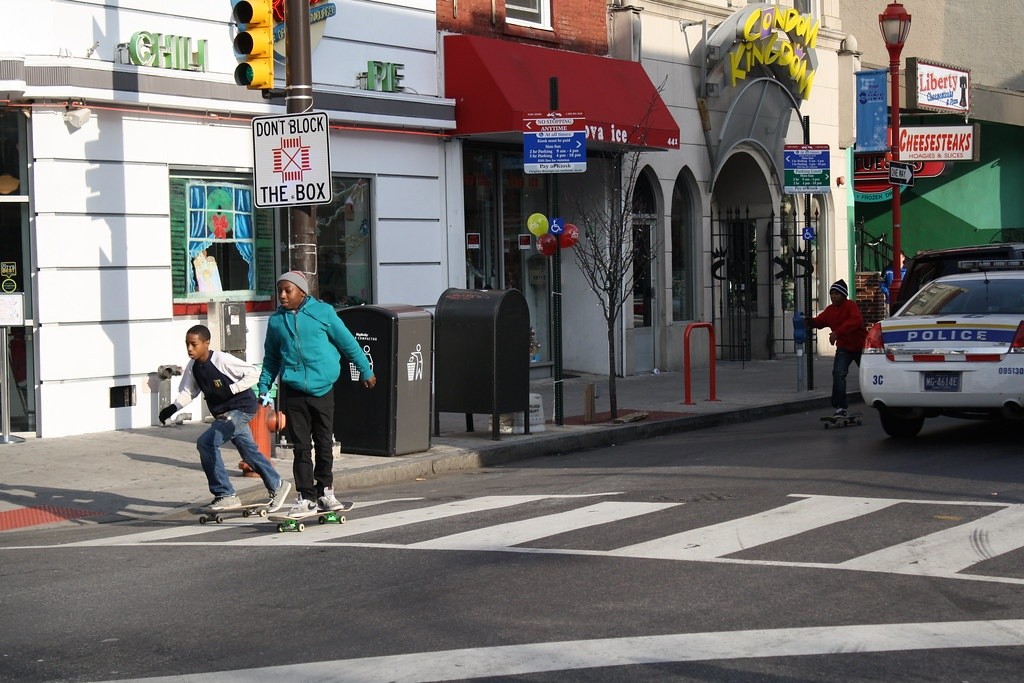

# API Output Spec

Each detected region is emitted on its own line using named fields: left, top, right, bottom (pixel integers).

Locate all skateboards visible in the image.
left=267, top=501, right=355, bottom=532
left=819, top=412, right=864, bottom=429
left=187, top=497, right=273, bottom=525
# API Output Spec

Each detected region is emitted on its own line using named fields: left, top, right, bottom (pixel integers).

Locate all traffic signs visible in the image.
left=889, top=160, right=914, bottom=186
left=522, top=110, right=587, bottom=174
left=782, top=144, right=831, bottom=195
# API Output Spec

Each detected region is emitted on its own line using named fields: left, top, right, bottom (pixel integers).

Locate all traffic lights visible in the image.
left=233, top=0, right=275, bottom=93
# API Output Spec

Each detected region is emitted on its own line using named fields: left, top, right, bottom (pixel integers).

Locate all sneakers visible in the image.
left=208, top=494, right=241, bottom=509
left=834, top=408, right=848, bottom=418
left=267, top=479, right=291, bottom=513
left=288, top=493, right=317, bottom=517
left=318, top=487, right=344, bottom=511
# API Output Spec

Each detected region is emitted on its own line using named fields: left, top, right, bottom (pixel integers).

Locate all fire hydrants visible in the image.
left=238, top=401, right=286, bottom=477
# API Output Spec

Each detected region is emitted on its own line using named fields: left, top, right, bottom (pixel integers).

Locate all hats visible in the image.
left=276, top=270, right=308, bottom=296
left=830, top=279, right=848, bottom=298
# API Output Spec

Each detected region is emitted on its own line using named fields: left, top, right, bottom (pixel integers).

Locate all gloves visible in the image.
left=804, top=317, right=813, bottom=328
left=204, top=385, right=233, bottom=405
left=159, top=404, right=177, bottom=425
left=828, top=332, right=836, bottom=345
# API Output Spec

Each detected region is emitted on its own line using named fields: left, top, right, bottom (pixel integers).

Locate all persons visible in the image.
left=158, top=325, right=290, bottom=512
left=803, top=279, right=869, bottom=418
left=879, top=249, right=908, bottom=318
left=256, top=270, right=376, bottom=518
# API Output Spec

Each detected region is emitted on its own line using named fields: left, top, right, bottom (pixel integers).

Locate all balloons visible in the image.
left=527, top=213, right=579, bottom=257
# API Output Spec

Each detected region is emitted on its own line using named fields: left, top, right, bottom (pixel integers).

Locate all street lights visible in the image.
left=877, top=0, right=912, bottom=310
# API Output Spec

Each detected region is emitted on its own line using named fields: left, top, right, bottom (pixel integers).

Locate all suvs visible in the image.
left=891, top=240, right=1024, bottom=319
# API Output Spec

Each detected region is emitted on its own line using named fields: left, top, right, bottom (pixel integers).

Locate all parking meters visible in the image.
left=793, top=311, right=807, bottom=393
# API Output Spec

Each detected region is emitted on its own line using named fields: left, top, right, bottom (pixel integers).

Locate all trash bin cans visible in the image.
left=433, top=286, right=531, bottom=443
left=333, top=304, right=435, bottom=456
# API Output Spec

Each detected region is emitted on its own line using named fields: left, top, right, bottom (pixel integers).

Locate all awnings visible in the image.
left=443, top=35, right=679, bottom=154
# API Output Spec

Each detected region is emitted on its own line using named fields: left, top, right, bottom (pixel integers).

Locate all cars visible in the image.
left=857, top=257, right=1024, bottom=440
